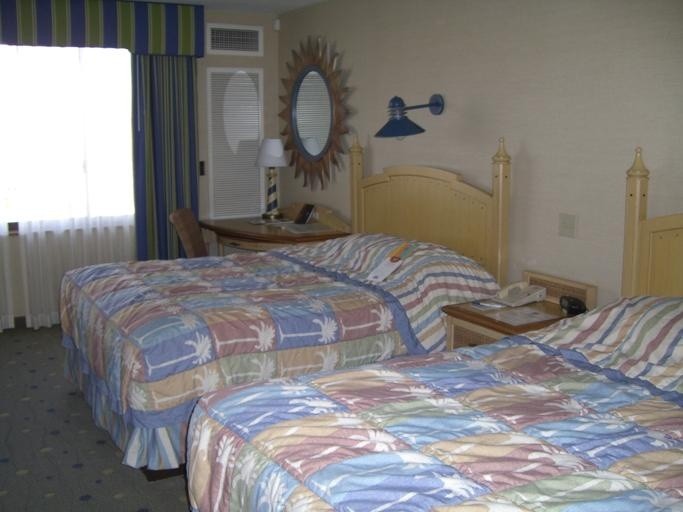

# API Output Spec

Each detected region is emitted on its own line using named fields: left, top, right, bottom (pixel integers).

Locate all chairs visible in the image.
left=169, top=206, right=206, bottom=258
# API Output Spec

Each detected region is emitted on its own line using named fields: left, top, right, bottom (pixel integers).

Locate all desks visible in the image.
left=198, top=201, right=351, bottom=257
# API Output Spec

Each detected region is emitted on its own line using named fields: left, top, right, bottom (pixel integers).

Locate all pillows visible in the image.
left=308, top=231, right=495, bottom=305
left=556, top=294, right=683, bottom=382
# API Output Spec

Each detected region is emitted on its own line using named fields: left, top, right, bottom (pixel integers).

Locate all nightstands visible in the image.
left=442, top=291, right=561, bottom=349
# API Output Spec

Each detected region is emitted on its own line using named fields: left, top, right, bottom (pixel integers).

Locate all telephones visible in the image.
left=492, top=281, right=547, bottom=307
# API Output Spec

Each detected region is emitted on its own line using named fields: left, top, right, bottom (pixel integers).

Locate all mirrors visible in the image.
left=277, top=33, right=350, bottom=194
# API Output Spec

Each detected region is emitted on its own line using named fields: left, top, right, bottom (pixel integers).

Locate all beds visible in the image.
left=56, top=131, right=512, bottom=482
left=182, top=146, right=683, bottom=512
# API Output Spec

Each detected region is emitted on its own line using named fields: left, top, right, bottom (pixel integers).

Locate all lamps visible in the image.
left=372, top=93, right=445, bottom=141
left=248, top=137, right=294, bottom=226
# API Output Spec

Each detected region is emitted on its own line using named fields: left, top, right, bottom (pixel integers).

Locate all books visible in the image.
left=279, top=222, right=336, bottom=234
